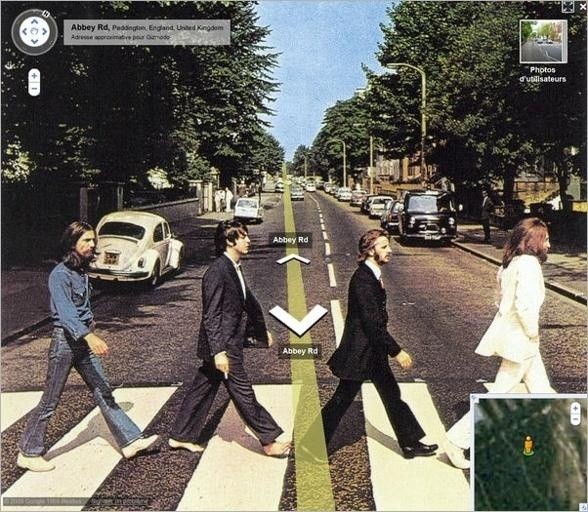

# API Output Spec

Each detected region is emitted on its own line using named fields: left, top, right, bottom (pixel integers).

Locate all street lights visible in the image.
left=353, top=124, right=373, bottom=193
left=299, top=154, right=308, bottom=177
left=325, top=140, right=346, bottom=188
left=387, top=61, right=427, bottom=188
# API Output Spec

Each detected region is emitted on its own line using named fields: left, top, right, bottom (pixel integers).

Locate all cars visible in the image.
left=532, top=36, right=553, bottom=44
left=381, top=200, right=404, bottom=236
left=275, top=174, right=395, bottom=219
left=85, top=210, right=186, bottom=288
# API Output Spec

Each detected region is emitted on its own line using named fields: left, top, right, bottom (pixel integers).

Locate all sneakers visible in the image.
left=121, top=435, right=158, bottom=459
left=442, top=440, right=469, bottom=470
left=17, top=453, right=54, bottom=473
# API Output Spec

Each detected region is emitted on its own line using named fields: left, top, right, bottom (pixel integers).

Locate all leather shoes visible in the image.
left=294, top=445, right=330, bottom=464
left=402, top=442, right=437, bottom=458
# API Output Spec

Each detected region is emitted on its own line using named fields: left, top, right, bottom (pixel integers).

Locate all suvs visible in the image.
left=234, top=198, right=264, bottom=223
left=398, top=188, right=458, bottom=244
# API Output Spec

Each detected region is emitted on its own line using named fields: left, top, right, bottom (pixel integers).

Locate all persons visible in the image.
left=479, top=189, right=495, bottom=245
left=167, top=219, right=295, bottom=459
left=297, top=228, right=439, bottom=466
left=442, top=216, right=561, bottom=469
left=15, top=218, right=162, bottom=473
left=213, top=186, right=234, bottom=213
left=223, top=371, right=230, bottom=380
